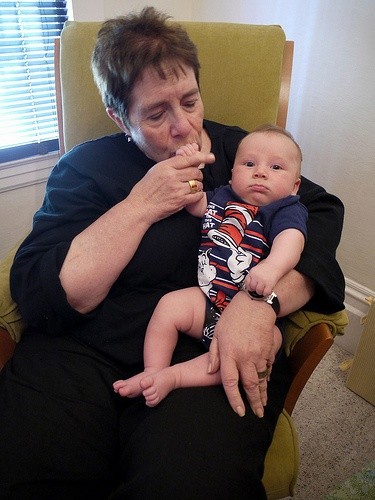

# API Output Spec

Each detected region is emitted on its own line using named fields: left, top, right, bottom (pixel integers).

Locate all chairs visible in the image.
left=0, top=21, right=348, bottom=500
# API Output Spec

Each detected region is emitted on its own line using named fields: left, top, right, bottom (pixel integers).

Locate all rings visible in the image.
left=189, top=180, right=198, bottom=194
left=257, top=368, right=268, bottom=379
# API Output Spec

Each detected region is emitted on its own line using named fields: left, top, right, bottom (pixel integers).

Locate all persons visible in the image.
left=112, top=122, right=309, bottom=407
left=0, top=6, right=346, bottom=500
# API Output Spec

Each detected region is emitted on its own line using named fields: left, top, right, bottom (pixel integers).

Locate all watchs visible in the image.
left=239, top=283, right=280, bottom=317
